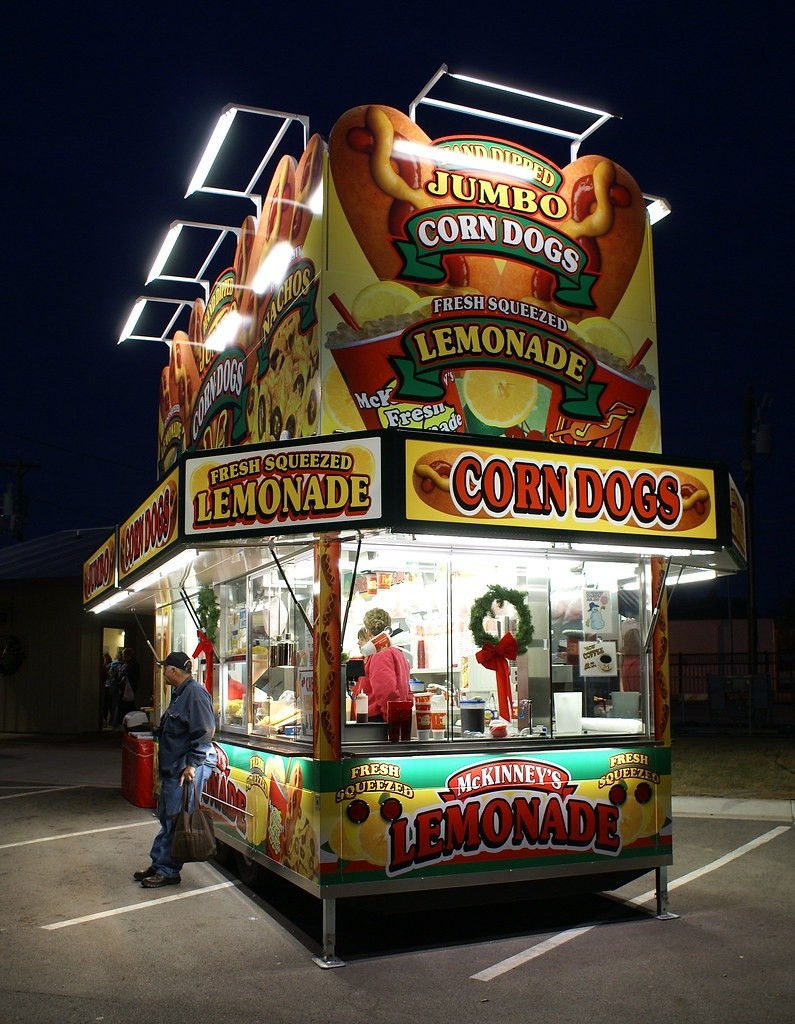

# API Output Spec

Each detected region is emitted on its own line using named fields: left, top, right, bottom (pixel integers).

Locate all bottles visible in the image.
left=355, top=687, right=368, bottom=723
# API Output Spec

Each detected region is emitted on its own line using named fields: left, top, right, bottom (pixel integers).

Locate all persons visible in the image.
left=132, top=651, right=216, bottom=888
left=349, top=607, right=415, bottom=723
left=102, top=647, right=141, bottom=728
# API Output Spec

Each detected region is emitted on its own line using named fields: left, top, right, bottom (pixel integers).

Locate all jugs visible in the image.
left=457, top=699, right=498, bottom=737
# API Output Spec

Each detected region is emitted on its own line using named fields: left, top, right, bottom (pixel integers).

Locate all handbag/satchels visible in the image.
left=171, top=769, right=218, bottom=864
left=120, top=676, right=136, bottom=702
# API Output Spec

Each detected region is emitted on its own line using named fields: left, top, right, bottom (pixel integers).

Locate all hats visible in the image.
left=156, top=650, right=193, bottom=673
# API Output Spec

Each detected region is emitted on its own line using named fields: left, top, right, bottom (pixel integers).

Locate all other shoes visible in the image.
left=134, top=868, right=156, bottom=881
left=141, top=873, right=182, bottom=888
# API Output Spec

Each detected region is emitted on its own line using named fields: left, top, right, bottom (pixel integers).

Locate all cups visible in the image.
left=414, top=692, right=446, bottom=740
left=362, top=632, right=393, bottom=657
left=598, top=654, right=613, bottom=670
left=387, top=701, right=413, bottom=741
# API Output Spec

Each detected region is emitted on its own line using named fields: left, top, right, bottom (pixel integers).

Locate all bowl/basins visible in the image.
left=409, top=681, right=425, bottom=693
